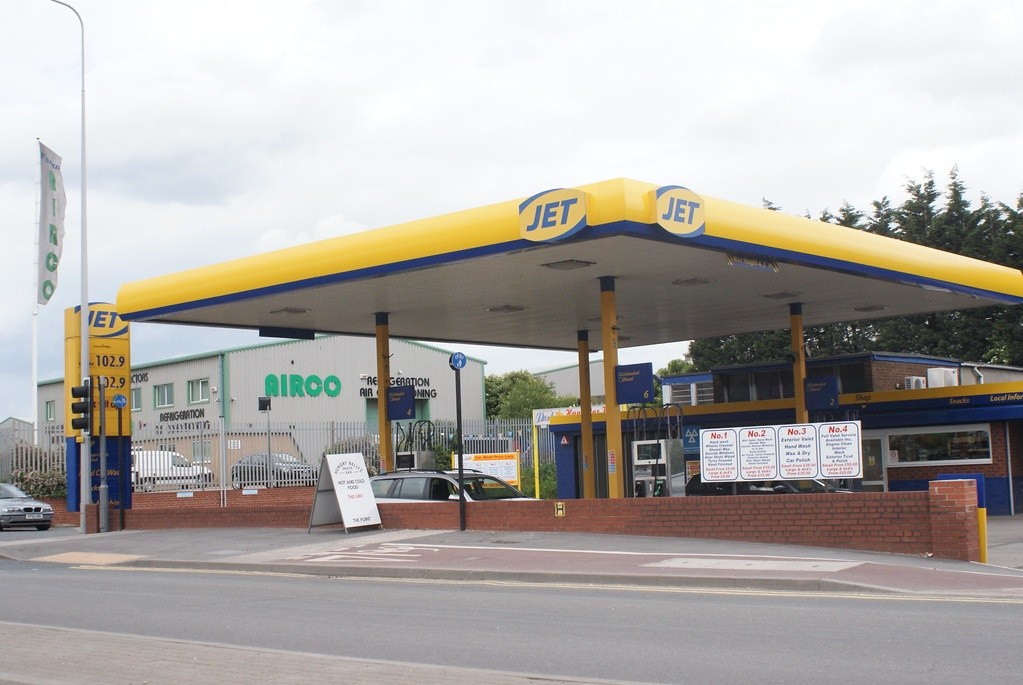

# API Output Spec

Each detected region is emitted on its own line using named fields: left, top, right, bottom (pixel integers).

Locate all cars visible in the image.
left=230, top=450, right=316, bottom=491
left=131, top=450, right=213, bottom=492
left=0, top=483, right=55, bottom=531
left=685, top=471, right=857, bottom=496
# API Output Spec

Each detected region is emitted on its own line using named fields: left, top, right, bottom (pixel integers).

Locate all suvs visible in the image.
left=370, top=468, right=543, bottom=503
